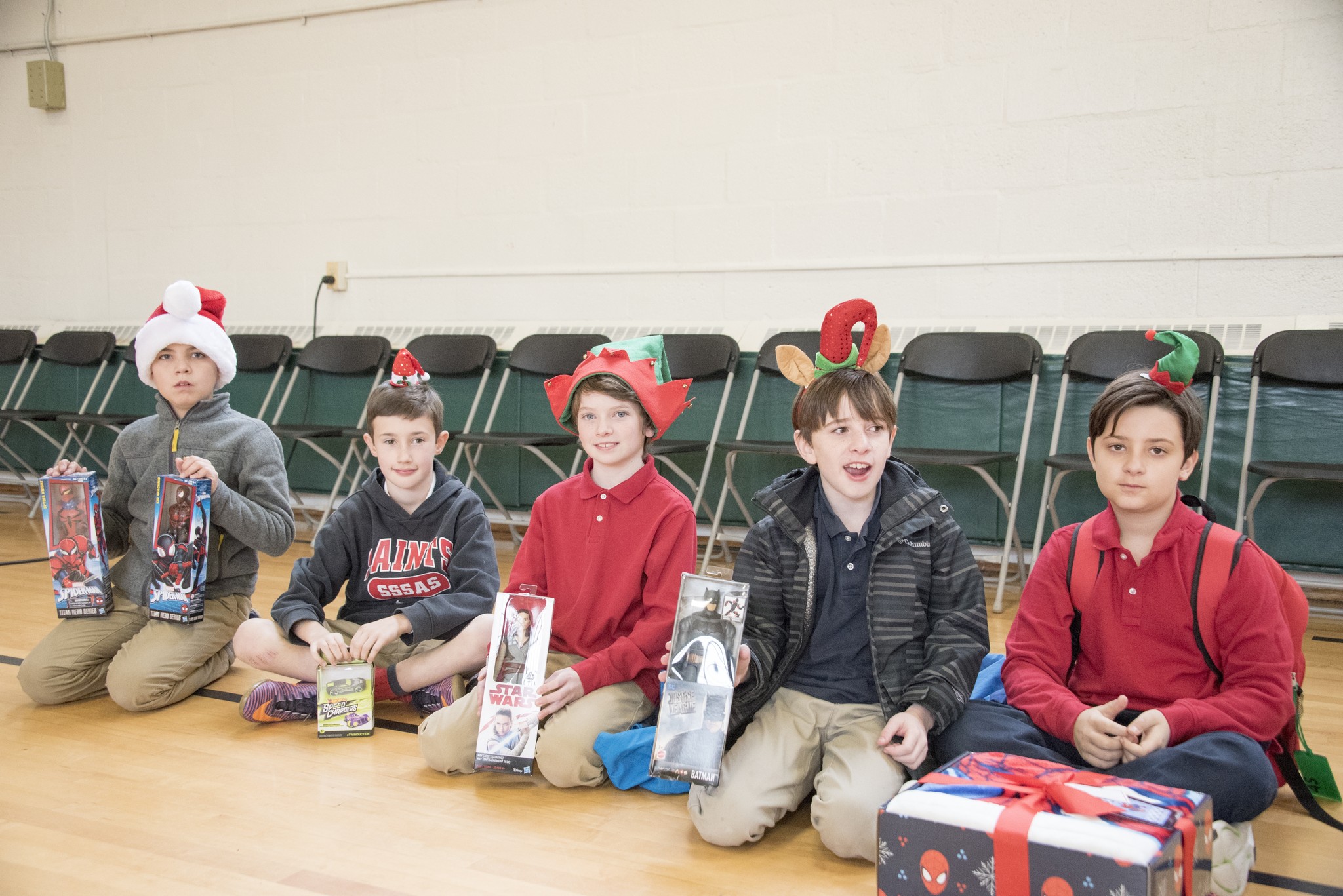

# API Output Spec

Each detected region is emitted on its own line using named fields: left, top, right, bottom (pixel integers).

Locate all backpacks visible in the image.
left=1065, top=493, right=1309, bottom=788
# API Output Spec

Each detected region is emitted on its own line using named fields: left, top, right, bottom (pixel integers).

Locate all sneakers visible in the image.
left=414, top=675, right=466, bottom=719
left=1208, top=820, right=1257, bottom=896
left=239, top=679, right=319, bottom=724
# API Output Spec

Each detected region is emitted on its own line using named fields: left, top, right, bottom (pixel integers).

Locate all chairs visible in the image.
left=0, top=330, right=1343, bottom=615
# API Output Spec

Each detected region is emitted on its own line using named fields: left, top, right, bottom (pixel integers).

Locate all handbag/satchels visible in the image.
left=592, top=724, right=690, bottom=794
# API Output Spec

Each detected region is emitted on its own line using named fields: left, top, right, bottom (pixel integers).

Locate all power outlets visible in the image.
left=326, top=260, right=347, bottom=294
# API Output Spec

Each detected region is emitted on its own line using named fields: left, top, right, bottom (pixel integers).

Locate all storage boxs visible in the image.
left=38, top=471, right=115, bottom=620
left=149, top=474, right=213, bottom=624
left=474, top=584, right=555, bottom=774
left=317, top=657, right=379, bottom=740
left=648, top=564, right=751, bottom=788
left=876, top=752, right=1213, bottom=896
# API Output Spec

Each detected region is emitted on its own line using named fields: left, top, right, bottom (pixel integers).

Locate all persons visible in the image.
left=417, top=336, right=698, bottom=786
left=18, top=281, right=296, bottom=713
left=930, top=331, right=1296, bottom=896
left=658, top=301, right=989, bottom=863
left=234, top=349, right=500, bottom=724
left=665, top=587, right=736, bottom=771
left=487, top=608, right=532, bottom=758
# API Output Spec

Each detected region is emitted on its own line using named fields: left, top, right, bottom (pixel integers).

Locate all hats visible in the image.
left=134, top=280, right=237, bottom=392
left=1139, top=329, right=1200, bottom=398
left=544, top=335, right=697, bottom=444
left=390, top=348, right=430, bottom=388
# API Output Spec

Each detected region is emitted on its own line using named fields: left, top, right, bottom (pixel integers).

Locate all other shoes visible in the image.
left=249, top=608, right=261, bottom=619
left=466, top=673, right=479, bottom=694
